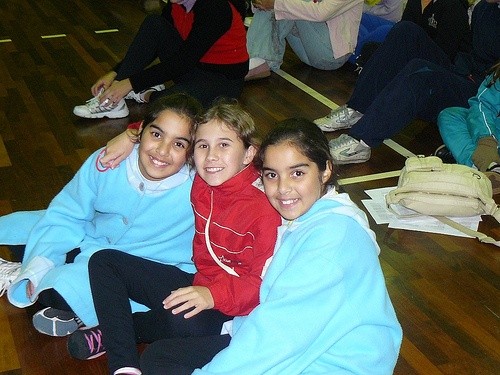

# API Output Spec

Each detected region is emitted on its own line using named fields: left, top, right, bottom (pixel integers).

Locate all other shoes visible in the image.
left=243, top=17, right=255, bottom=26
left=481, top=170, right=500, bottom=195
left=244, top=61, right=271, bottom=80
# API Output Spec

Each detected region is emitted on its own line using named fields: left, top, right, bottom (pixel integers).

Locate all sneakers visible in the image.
left=328, top=134, right=372, bottom=164
left=124, top=84, right=165, bottom=103
left=312, top=104, right=363, bottom=131
left=32, top=307, right=85, bottom=336
left=73, top=87, right=129, bottom=118
left=67, top=325, right=106, bottom=361
left=0, top=258, right=21, bottom=297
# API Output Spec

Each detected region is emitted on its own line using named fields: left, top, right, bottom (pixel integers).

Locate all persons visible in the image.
left=312, top=0, right=500, bottom=165
left=437, top=63, right=500, bottom=196
left=244, top=0, right=364, bottom=79
left=71, top=0, right=249, bottom=120
left=0, top=91, right=403, bottom=375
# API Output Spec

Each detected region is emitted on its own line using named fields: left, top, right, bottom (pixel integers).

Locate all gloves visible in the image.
left=471, top=136, right=500, bottom=171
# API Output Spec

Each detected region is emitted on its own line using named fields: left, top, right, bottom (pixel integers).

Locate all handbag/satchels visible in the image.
left=383, top=138, right=500, bottom=246
